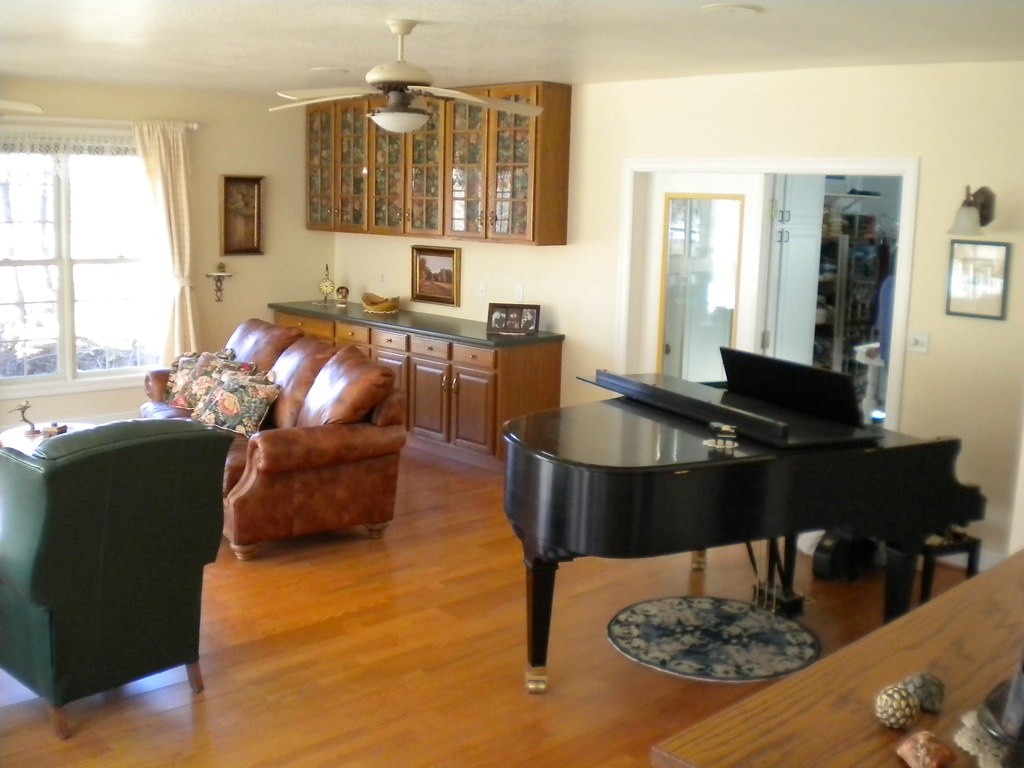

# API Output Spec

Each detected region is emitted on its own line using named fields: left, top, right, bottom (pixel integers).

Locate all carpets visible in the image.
left=606, top=593, right=821, bottom=685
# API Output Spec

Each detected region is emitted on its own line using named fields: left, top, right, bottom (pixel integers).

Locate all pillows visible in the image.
left=163, top=319, right=395, bottom=439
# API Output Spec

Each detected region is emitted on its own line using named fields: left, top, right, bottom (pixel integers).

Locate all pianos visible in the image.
left=500, top=346, right=988, bottom=694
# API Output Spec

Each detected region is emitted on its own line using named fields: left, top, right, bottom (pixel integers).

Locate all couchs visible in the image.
left=145, top=318, right=408, bottom=562
left=0, top=418, right=233, bottom=740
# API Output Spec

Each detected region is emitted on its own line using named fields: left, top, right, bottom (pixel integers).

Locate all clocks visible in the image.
left=313, top=264, right=334, bottom=304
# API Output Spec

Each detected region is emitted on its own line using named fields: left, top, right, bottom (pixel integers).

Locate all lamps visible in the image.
left=959, top=185, right=996, bottom=225
left=368, top=93, right=430, bottom=133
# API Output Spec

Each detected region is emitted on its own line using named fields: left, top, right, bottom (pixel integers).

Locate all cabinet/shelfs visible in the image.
left=302, top=79, right=570, bottom=245
left=815, top=233, right=849, bottom=374
left=272, top=310, right=559, bottom=472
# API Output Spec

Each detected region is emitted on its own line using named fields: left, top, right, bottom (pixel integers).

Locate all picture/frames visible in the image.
left=218, top=174, right=265, bottom=256
left=946, top=239, right=1010, bottom=319
left=488, top=302, right=541, bottom=336
left=411, top=244, right=460, bottom=307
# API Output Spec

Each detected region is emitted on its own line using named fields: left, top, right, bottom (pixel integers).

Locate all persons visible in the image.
left=878, top=242, right=899, bottom=370
left=492, top=310, right=535, bottom=329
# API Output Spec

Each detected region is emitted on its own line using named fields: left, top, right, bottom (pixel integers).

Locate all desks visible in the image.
left=0, top=422, right=96, bottom=458
left=651, top=551, right=1023, bottom=768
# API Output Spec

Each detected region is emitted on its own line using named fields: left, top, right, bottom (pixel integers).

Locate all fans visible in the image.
left=267, top=18, right=543, bottom=116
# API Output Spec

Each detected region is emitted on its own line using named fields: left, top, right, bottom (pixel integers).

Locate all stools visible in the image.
left=923, top=532, right=980, bottom=603
